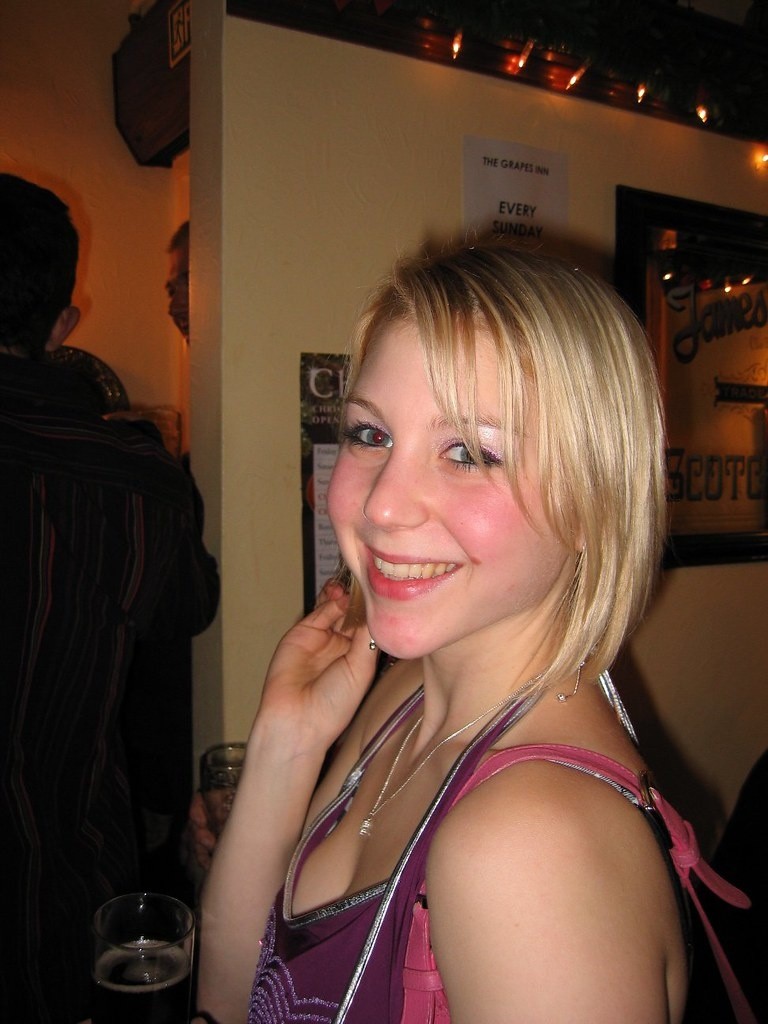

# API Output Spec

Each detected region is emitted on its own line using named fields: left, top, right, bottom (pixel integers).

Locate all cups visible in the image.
left=89, top=894, right=196, bottom=1024
left=199, top=744, right=246, bottom=839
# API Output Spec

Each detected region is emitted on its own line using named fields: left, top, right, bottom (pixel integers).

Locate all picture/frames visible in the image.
left=614, top=184, right=768, bottom=570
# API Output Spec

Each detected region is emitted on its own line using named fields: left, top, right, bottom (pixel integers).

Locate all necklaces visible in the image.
left=359, top=661, right=587, bottom=836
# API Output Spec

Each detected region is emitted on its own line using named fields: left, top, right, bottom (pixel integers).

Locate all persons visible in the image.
left=195, top=216, right=754, bottom=1024
left=0, top=176, right=217, bottom=1024
